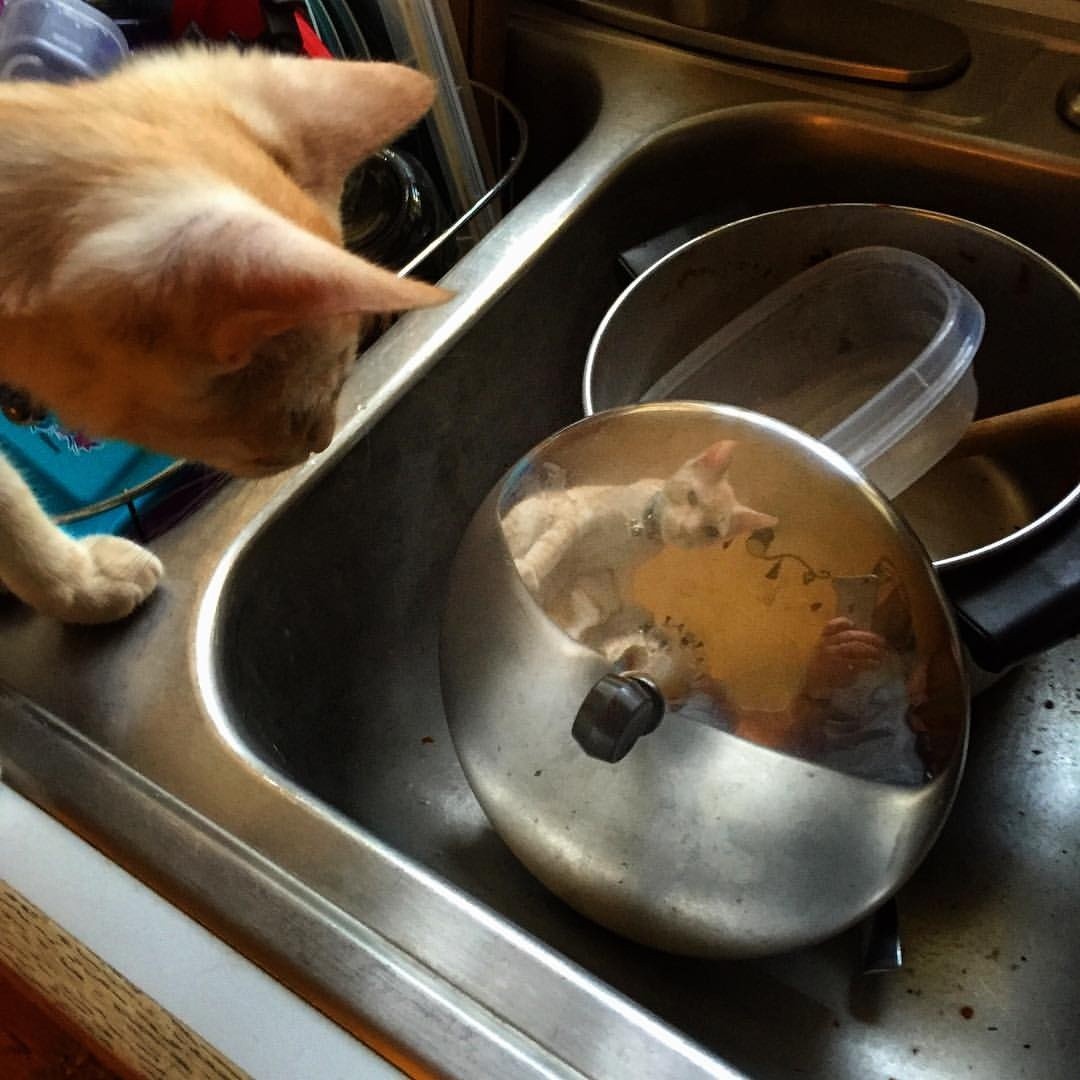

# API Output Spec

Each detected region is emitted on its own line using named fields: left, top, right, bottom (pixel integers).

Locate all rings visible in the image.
left=847, top=658, right=855, bottom=671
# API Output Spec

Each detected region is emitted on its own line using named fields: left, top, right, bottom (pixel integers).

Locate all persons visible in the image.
left=811, top=618, right=921, bottom=789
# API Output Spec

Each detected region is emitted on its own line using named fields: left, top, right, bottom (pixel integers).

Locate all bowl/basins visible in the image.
left=633, top=247, right=987, bottom=501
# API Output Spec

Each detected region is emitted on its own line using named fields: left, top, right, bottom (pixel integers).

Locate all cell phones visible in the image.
left=836, top=574, right=878, bottom=647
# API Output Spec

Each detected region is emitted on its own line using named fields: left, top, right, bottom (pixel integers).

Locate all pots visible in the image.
left=579, top=197, right=1080, bottom=704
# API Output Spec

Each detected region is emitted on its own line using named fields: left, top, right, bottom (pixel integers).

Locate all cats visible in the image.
left=502, top=437, right=779, bottom=620
left=0, top=42, right=461, bottom=625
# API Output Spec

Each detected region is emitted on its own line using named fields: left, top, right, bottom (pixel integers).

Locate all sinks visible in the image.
left=0, top=2, right=609, bottom=689
left=163, top=91, right=1080, bottom=1080
